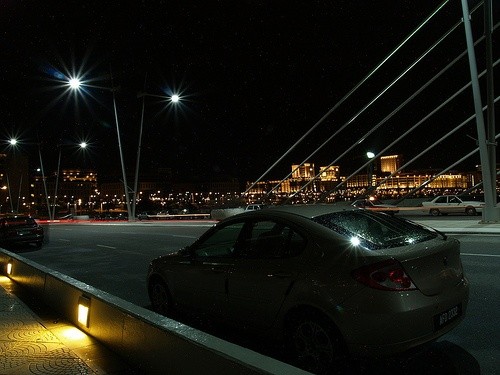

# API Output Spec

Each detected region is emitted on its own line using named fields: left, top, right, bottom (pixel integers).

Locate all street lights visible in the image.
left=366, top=151, right=375, bottom=190
left=9, top=139, right=87, bottom=221
left=68, top=76, right=182, bottom=221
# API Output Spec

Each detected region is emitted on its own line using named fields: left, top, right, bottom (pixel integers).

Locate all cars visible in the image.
left=142, top=201, right=478, bottom=375
left=0, top=217, right=45, bottom=251
left=351, top=199, right=400, bottom=217
left=421, top=194, right=485, bottom=217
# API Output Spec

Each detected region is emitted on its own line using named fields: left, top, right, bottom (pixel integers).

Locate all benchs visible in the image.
left=149, top=214, right=210, bottom=220
